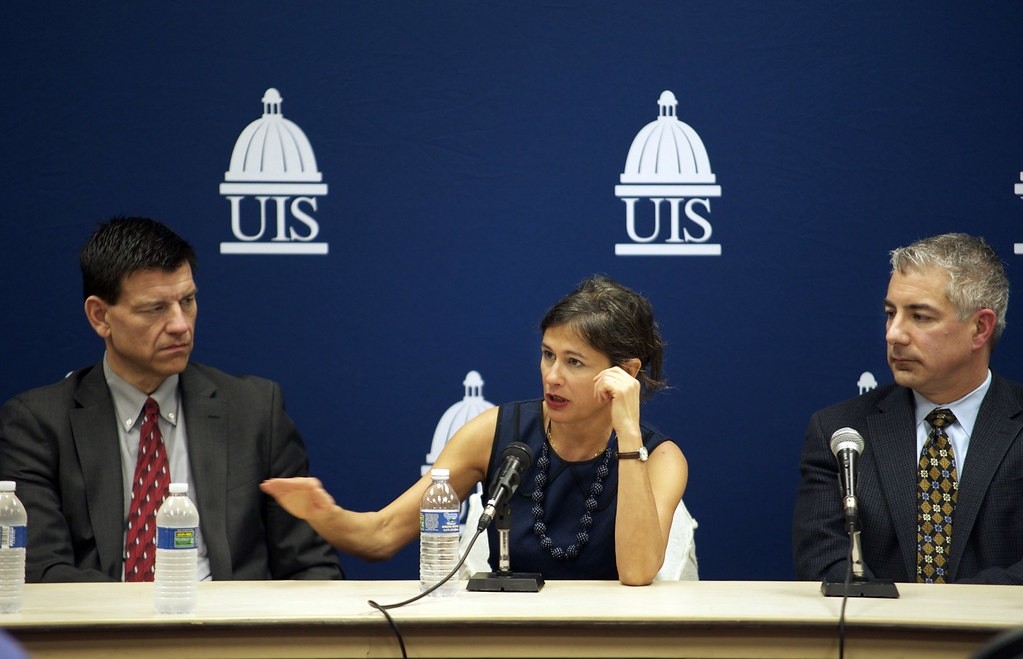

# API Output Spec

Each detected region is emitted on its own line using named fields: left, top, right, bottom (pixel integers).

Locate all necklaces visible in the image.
left=529, top=418, right=613, bottom=560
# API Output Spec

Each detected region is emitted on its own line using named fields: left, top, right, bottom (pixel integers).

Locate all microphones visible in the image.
left=478, top=442, right=533, bottom=532
left=830, top=428, right=864, bottom=526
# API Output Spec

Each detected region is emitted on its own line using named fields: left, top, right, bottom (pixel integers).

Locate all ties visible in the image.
left=916, top=408, right=958, bottom=583
left=124, top=398, right=172, bottom=582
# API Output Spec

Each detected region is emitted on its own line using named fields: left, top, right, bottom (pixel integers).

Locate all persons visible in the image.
left=0, top=215, right=343, bottom=584
left=260, top=278, right=688, bottom=586
left=791, top=233, right=1023, bottom=585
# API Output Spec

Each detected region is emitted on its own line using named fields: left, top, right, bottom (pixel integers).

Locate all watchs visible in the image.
left=614, top=446, right=648, bottom=463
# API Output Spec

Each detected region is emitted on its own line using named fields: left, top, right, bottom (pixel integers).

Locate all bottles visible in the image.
left=156, top=483, right=199, bottom=614
left=420, top=469, right=459, bottom=596
left=0, top=480, right=27, bottom=612
